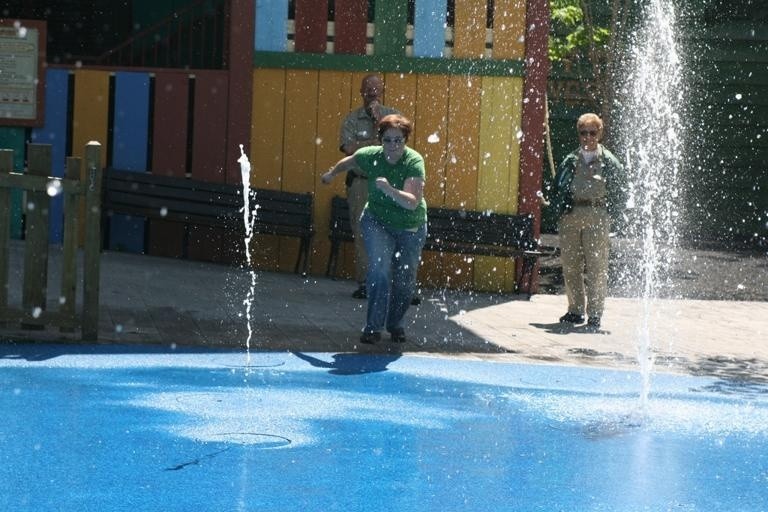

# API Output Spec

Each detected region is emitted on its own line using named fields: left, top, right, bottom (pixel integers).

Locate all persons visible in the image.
left=320, top=113, right=428, bottom=344
left=339, top=75, right=421, bottom=305
left=552, top=113, right=627, bottom=326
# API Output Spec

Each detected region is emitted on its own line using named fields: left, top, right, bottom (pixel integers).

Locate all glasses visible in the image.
left=580, top=130, right=598, bottom=136
left=381, top=135, right=409, bottom=144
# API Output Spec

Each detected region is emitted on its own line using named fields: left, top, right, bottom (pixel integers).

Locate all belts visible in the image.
left=573, top=201, right=605, bottom=208
left=353, top=174, right=368, bottom=181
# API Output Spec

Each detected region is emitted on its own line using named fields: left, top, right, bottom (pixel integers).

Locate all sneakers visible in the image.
left=361, top=332, right=382, bottom=344
left=560, top=313, right=586, bottom=323
left=586, top=317, right=601, bottom=327
left=353, top=286, right=367, bottom=299
left=392, top=330, right=406, bottom=344
left=411, top=297, right=420, bottom=306
